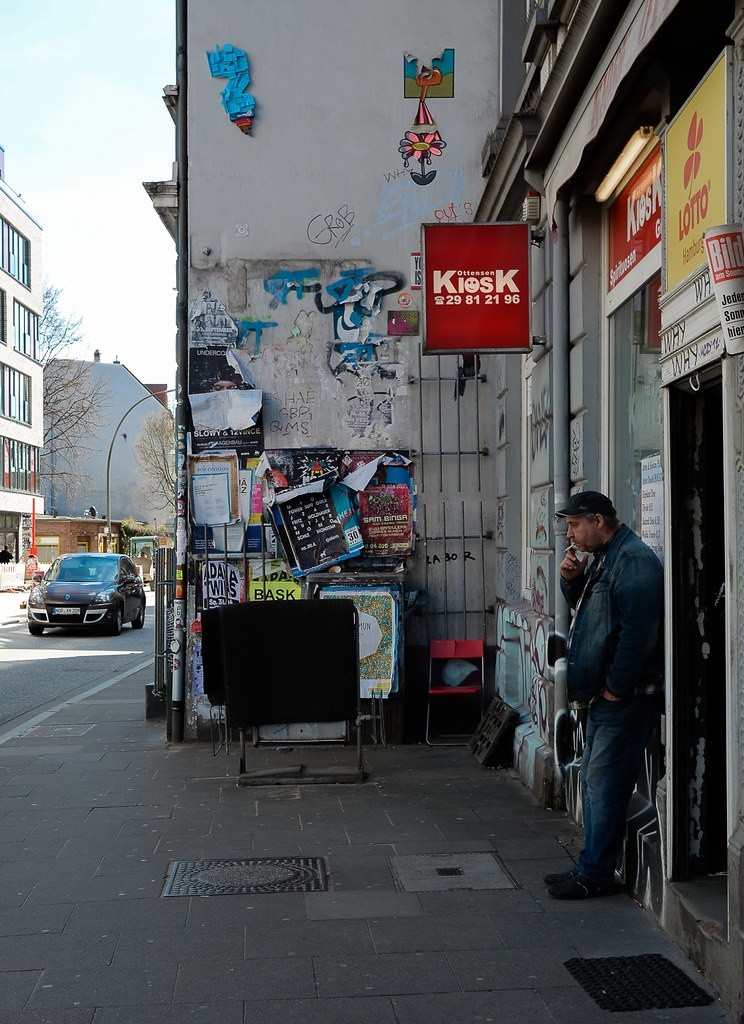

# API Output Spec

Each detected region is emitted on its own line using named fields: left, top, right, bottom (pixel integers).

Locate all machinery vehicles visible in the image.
left=129, top=536, right=159, bottom=591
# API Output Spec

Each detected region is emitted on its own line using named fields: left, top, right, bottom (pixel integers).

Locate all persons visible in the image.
left=543, top=491, right=664, bottom=900
left=0, top=545, right=13, bottom=563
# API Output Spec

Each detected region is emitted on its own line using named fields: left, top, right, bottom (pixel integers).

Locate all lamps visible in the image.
left=594, top=127, right=660, bottom=211
left=89, top=506, right=98, bottom=519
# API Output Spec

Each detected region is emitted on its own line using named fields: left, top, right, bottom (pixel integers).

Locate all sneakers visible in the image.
left=543, top=868, right=615, bottom=900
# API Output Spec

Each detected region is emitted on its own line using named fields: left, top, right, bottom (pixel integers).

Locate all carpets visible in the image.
left=562, top=953, right=716, bottom=1013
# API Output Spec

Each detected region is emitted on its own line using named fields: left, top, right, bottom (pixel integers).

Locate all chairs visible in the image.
left=424, top=639, right=485, bottom=746
left=65, top=568, right=90, bottom=579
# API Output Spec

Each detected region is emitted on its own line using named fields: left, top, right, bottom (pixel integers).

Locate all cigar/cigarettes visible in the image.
left=563, top=543, right=575, bottom=552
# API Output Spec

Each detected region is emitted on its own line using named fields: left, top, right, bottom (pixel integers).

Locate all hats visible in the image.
left=211, top=366, right=241, bottom=388
left=555, top=491, right=613, bottom=517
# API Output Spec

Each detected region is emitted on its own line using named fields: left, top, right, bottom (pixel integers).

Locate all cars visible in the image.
left=26, top=553, right=146, bottom=635
